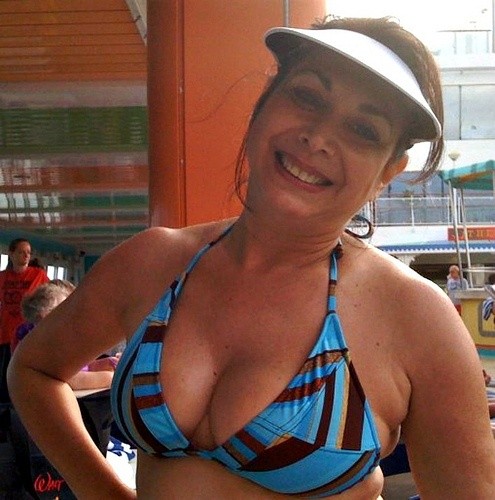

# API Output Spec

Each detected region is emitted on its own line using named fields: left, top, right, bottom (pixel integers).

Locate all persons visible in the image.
left=7, top=18, right=495, bottom=499
left=0, top=238, right=126, bottom=500
left=446, top=264, right=469, bottom=317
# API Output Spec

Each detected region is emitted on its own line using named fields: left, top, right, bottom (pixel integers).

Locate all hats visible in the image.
left=263, top=26, right=442, bottom=151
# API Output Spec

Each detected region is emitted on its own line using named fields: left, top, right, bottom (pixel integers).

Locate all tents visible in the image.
left=437, top=160, right=494, bottom=290
